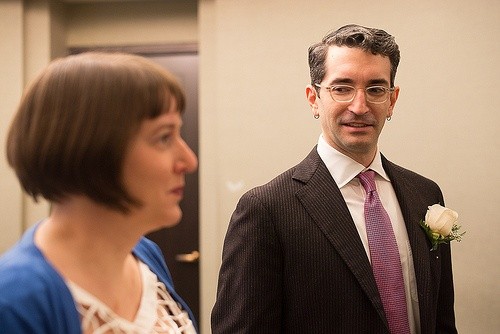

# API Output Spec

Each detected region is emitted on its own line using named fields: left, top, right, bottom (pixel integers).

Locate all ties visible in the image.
left=359, top=171, right=412, bottom=334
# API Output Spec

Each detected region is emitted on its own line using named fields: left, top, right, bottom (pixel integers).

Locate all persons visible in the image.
left=211, top=24, right=459, bottom=334
left=0, top=53, right=199, bottom=334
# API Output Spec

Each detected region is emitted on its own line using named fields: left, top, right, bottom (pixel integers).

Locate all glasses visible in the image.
left=314, top=82, right=394, bottom=104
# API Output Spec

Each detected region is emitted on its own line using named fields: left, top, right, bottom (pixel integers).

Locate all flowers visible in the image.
left=418, top=203, right=467, bottom=252
